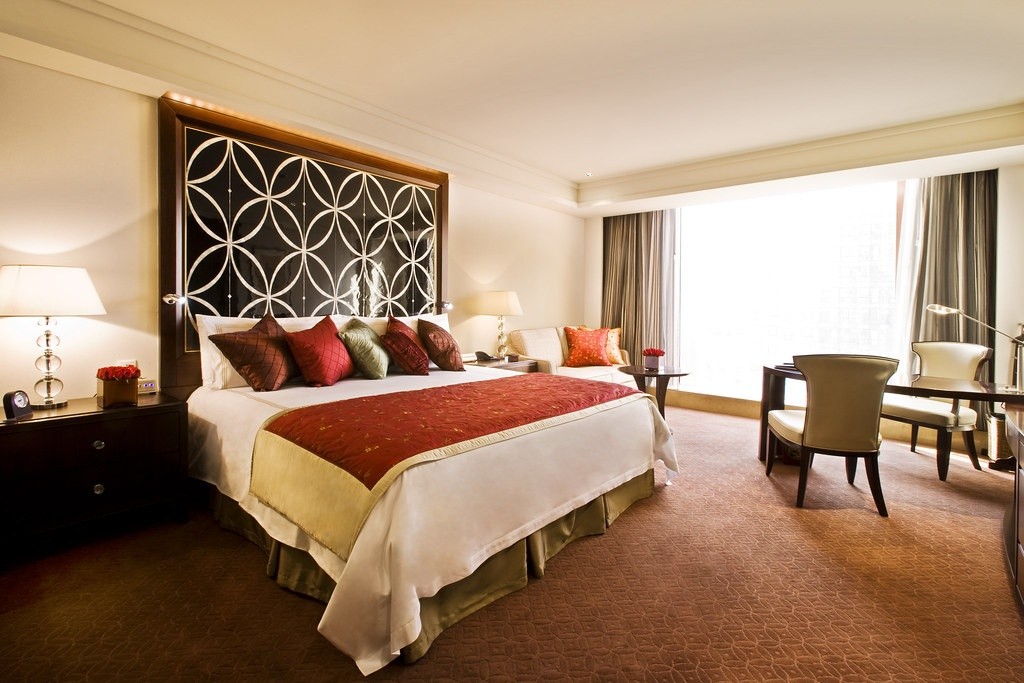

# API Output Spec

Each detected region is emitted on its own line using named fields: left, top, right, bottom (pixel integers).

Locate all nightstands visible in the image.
left=0, top=392, right=190, bottom=573
left=468, top=358, right=538, bottom=373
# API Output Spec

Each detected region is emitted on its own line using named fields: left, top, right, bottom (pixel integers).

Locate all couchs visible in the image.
left=508, top=325, right=638, bottom=390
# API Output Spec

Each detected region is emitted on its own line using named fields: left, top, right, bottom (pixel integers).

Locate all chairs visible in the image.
left=880, top=340, right=993, bottom=481
left=765, top=353, right=900, bottom=517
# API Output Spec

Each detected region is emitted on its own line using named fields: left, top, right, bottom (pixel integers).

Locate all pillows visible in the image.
left=564, top=327, right=612, bottom=366
left=380, top=315, right=429, bottom=375
left=418, top=318, right=466, bottom=371
left=208, top=314, right=299, bottom=391
left=337, top=319, right=394, bottom=380
left=580, top=326, right=625, bottom=364
left=282, top=315, right=358, bottom=387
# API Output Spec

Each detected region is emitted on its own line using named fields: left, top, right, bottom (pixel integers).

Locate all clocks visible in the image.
left=3, top=390, right=33, bottom=422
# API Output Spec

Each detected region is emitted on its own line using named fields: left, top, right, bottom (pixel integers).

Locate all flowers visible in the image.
left=97, top=365, right=141, bottom=381
left=642, top=348, right=665, bottom=356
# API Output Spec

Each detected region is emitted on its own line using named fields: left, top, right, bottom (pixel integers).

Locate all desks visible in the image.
left=617, top=365, right=689, bottom=419
left=758, top=364, right=1024, bottom=461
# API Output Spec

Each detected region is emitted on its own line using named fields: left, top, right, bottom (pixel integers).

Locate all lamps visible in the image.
left=476, top=291, right=523, bottom=360
left=927, top=303, right=1024, bottom=346
left=0, top=263, right=106, bottom=409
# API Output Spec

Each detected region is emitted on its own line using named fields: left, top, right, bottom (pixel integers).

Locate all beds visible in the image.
left=158, top=93, right=683, bottom=675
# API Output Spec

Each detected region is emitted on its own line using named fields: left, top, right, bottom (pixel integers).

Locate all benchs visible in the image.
left=876, top=391, right=980, bottom=480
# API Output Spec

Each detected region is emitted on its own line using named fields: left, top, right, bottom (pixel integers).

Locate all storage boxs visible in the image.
left=986, top=413, right=1013, bottom=460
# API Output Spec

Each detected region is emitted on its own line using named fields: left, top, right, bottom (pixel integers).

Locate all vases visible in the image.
left=645, top=356, right=659, bottom=369
left=96, top=378, right=138, bottom=408
